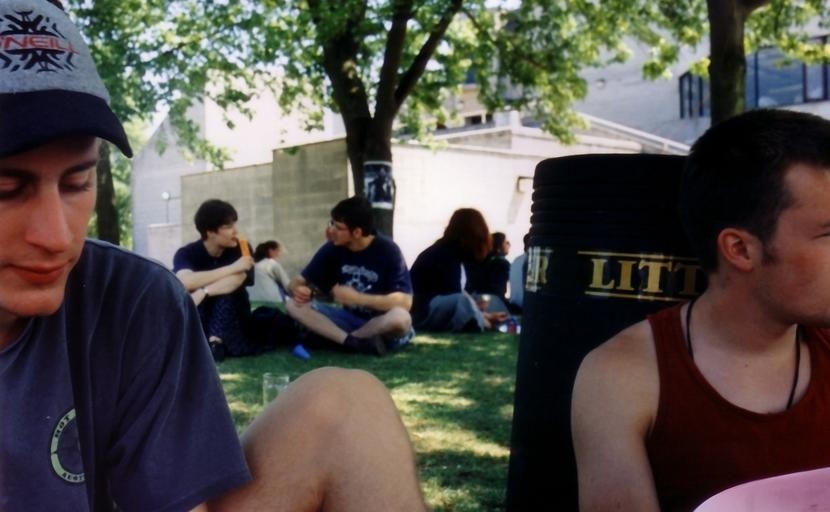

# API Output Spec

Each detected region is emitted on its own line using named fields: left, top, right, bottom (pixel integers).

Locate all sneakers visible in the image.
left=208, top=341, right=224, bottom=361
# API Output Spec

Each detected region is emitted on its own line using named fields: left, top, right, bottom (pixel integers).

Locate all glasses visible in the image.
left=328, top=220, right=346, bottom=231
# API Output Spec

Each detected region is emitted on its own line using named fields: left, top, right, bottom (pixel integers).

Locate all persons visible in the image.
left=173, top=197, right=256, bottom=365
left=247, top=240, right=290, bottom=311
left=285, top=196, right=413, bottom=357
left=1, top=0, right=428, bottom=512
left=486, top=232, right=511, bottom=297
left=409, top=207, right=507, bottom=334
left=509, top=234, right=529, bottom=312
left=569, top=108, right=830, bottom=512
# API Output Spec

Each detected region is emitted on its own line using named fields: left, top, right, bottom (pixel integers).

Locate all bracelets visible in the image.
left=201, top=285, right=208, bottom=298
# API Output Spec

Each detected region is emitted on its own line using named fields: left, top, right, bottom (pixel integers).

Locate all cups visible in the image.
left=261, top=372, right=290, bottom=403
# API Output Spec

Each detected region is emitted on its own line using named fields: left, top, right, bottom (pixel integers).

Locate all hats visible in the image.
left=0, top=0, right=134, bottom=163
